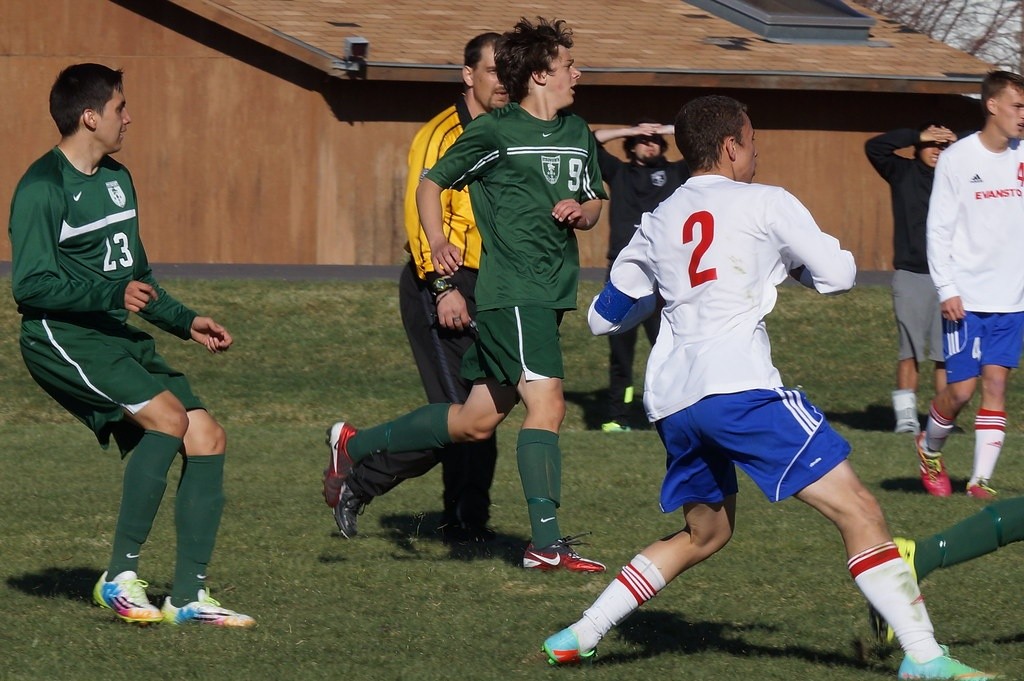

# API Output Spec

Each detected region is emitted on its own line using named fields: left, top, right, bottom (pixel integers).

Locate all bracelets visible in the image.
left=437, top=286, right=457, bottom=304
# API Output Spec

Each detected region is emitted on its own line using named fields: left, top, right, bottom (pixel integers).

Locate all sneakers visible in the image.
left=541, top=627, right=599, bottom=667
left=600, top=422, right=631, bottom=434
left=333, top=480, right=365, bottom=539
left=319, top=421, right=361, bottom=506
left=869, top=538, right=917, bottom=646
left=89, top=571, right=164, bottom=624
left=914, top=432, right=950, bottom=495
left=159, top=586, right=258, bottom=631
left=897, top=641, right=988, bottom=681
left=895, top=423, right=921, bottom=435
left=524, top=531, right=607, bottom=575
left=964, top=479, right=996, bottom=502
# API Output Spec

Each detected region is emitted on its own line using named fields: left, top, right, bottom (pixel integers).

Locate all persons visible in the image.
left=865, top=121, right=975, bottom=432
left=7, top=63, right=258, bottom=629
left=334, top=32, right=511, bottom=541
left=320, top=12, right=611, bottom=571
left=870, top=497, right=1024, bottom=642
left=540, top=95, right=1002, bottom=680
left=593, top=122, right=695, bottom=432
left=913, top=68, right=1024, bottom=498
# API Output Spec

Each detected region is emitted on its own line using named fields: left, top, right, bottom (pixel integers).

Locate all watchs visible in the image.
left=431, top=277, right=456, bottom=296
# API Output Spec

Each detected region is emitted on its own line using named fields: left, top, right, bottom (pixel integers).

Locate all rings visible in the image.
left=453, top=317, right=460, bottom=320
